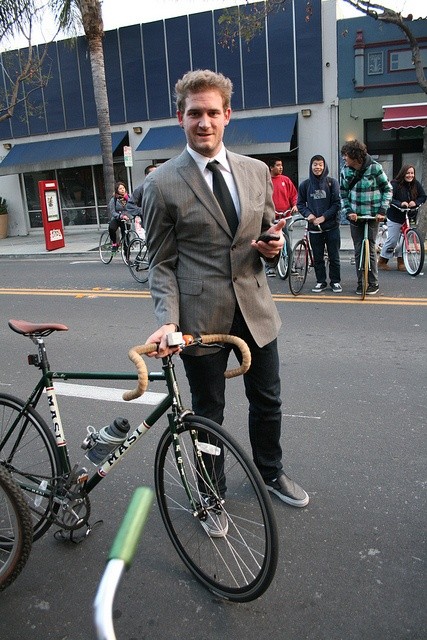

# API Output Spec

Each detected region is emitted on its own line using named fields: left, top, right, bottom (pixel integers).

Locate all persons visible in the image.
left=126, top=165, right=158, bottom=228
left=108, top=183, right=136, bottom=267
left=265, top=156, right=299, bottom=277
left=378, top=165, right=427, bottom=272
left=142, top=69, right=310, bottom=538
left=295, top=155, right=342, bottom=292
left=340, top=140, right=393, bottom=294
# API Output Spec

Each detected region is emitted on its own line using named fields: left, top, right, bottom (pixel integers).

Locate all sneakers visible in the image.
left=126, top=260, right=134, bottom=267
left=355, top=286, right=363, bottom=295
left=312, top=282, right=327, bottom=292
left=264, top=472, right=311, bottom=509
left=200, top=505, right=230, bottom=538
left=290, top=268, right=300, bottom=276
left=330, top=282, right=343, bottom=293
left=112, top=244, right=119, bottom=252
left=366, top=283, right=380, bottom=294
left=266, top=268, right=277, bottom=277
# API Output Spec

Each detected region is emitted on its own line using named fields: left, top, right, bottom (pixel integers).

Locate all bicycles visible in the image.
left=347, top=215, right=386, bottom=300
left=273, top=210, right=291, bottom=280
left=126, top=238, right=149, bottom=283
left=375, top=202, right=424, bottom=276
left=98, top=220, right=141, bottom=267
left=0, top=320, right=277, bottom=602
left=287, top=218, right=328, bottom=296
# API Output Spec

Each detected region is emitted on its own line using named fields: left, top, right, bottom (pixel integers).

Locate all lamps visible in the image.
left=301, top=109, right=311, bottom=117
left=3, top=144, right=11, bottom=150
left=133, top=127, right=142, bottom=133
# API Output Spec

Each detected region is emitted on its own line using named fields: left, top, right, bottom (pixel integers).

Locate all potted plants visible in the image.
left=0, top=196, right=8, bottom=238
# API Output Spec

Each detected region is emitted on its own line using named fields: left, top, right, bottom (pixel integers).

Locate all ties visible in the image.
left=206, top=160, right=240, bottom=239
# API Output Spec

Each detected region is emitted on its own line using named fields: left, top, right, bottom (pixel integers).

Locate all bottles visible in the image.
left=84, top=417, right=130, bottom=466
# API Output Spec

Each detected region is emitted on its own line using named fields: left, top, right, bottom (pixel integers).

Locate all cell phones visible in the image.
left=255, top=233, right=280, bottom=242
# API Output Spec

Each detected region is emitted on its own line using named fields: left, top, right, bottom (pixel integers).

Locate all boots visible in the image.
left=397, top=257, right=407, bottom=272
left=378, top=256, right=392, bottom=271
